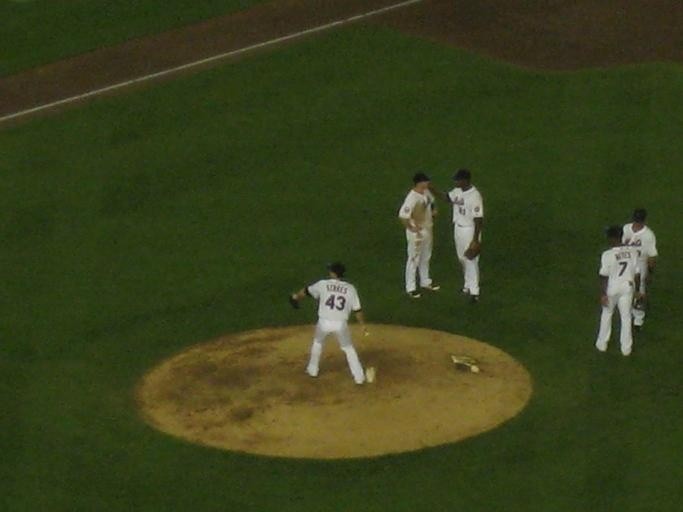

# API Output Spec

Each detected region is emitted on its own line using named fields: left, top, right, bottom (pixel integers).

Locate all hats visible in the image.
left=607, top=226, right=624, bottom=238
left=413, top=173, right=430, bottom=184
left=631, top=208, right=647, bottom=223
left=453, top=169, right=472, bottom=180
left=326, top=262, right=345, bottom=274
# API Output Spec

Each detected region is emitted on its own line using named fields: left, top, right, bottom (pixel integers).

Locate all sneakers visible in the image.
left=458, top=288, right=480, bottom=303
left=408, top=289, right=421, bottom=299
left=424, top=282, right=441, bottom=291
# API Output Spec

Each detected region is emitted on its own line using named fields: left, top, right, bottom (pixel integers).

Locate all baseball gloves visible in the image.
left=464, top=244, right=480, bottom=259
left=289, top=296, right=298, bottom=309
left=634, top=296, right=646, bottom=310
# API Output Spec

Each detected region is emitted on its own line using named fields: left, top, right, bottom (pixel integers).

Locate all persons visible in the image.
left=593, top=224, right=642, bottom=357
left=621, top=207, right=660, bottom=333
left=397, top=170, right=441, bottom=299
left=428, top=167, right=484, bottom=304
left=289, top=260, right=367, bottom=388
